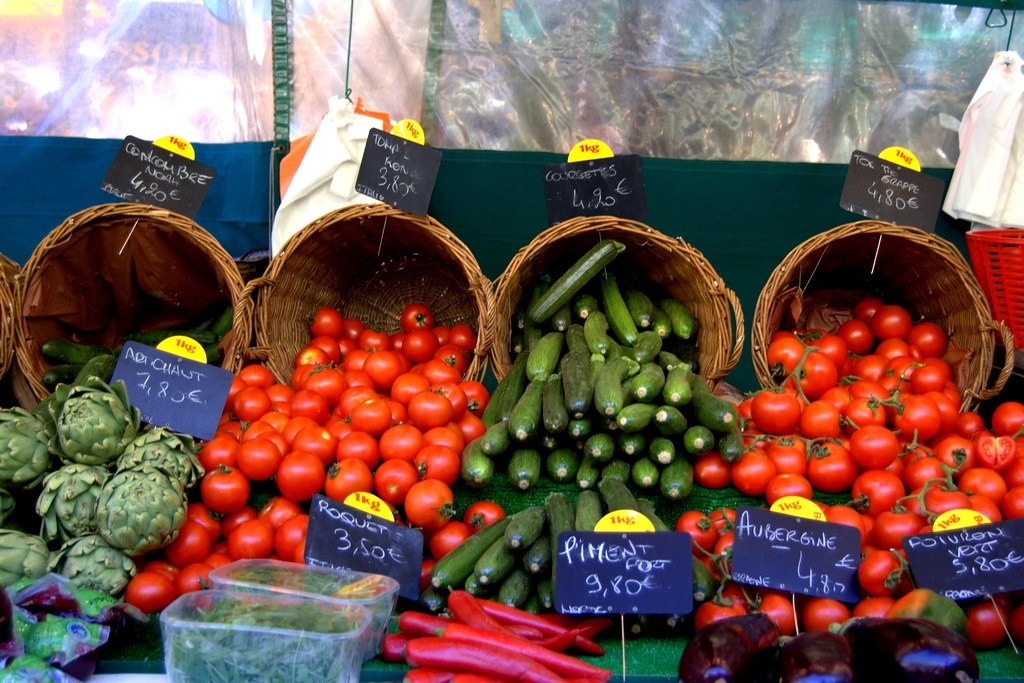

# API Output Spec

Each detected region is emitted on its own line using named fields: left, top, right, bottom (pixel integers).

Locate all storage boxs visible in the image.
left=11, top=206, right=253, bottom=409
left=158, top=594, right=369, bottom=682
left=210, top=559, right=401, bottom=667
left=966, top=228, right=1022, bottom=352
left=750, top=222, right=1013, bottom=420
left=232, top=205, right=497, bottom=390
left=484, top=219, right=744, bottom=396
left=0, top=251, right=21, bottom=376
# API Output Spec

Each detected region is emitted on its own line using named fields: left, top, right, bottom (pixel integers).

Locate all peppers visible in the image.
left=381, top=587, right=611, bottom=682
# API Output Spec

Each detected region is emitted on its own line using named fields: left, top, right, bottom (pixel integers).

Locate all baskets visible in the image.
left=487, top=217, right=745, bottom=395
left=750, top=221, right=1015, bottom=414
left=965, top=228, right=1023, bottom=352
left=233, top=204, right=496, bottom=385
left=1, top=202, right=255, bottom=404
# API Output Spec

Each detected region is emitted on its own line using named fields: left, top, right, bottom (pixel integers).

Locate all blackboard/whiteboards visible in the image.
left=101, top=135, right=216, bottom=221
left=901, top=517, right=1024, bottom=599
left=731, top=506, right=862, bottom=605
left=354, top=128, right=443, bottom=218
left=544, top=154, right=647, bottom=230
left=303, top=493, right=424, bottom=601
left=554, top=531, right=694, bottom=615
left=109, top=340, right=234, bottom=441
left=840, top=149, right=945, bottom=235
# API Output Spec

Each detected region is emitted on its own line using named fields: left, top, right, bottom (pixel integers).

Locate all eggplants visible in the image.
left=836, top=617, right=979, bottom=682
left=678, top=611, right=779, bottom=683
left=776, top=629, right=853, bottom=683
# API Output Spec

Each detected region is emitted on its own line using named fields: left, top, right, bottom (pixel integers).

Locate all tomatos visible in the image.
left=125, top=301, right=503, bottom=611
left=675, top=296, right=1023, bottom=644
